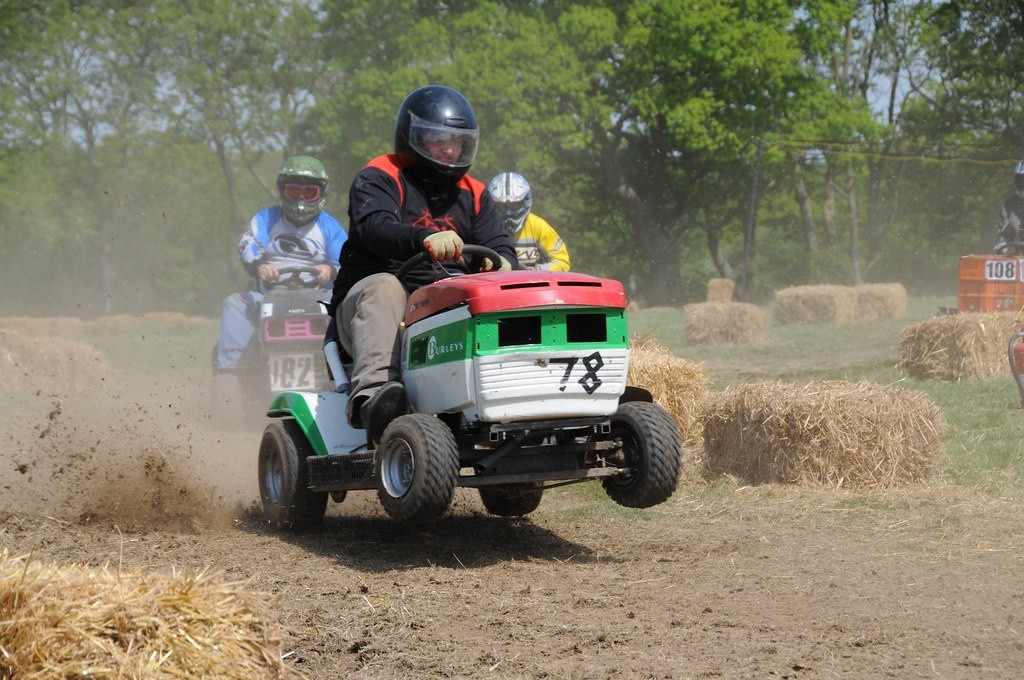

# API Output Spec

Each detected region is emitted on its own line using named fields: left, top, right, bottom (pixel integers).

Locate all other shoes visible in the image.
left=358, top=382, right=408, bottom=451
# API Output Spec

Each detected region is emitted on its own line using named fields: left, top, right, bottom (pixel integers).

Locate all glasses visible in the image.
left=283, top=184, right=320, bottom=201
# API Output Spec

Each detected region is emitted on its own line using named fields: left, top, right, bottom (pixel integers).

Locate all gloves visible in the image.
left=478, top=255, right=512, bottom=272
left=422, top=230, right=465, bottom=261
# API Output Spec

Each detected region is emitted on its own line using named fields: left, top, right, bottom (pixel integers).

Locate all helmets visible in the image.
left=276, top=155, right=329, bottom=224
left=394, top=84, right=480, bottom=184
left=486, top=172, right=533, bottom=235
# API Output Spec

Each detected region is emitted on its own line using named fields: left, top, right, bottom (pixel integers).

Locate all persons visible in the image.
left=994, top=159, right=1024, bottom=255
left=217, top=154, right=348, bottom=401
left=487, top=172, right=571, bottom=272
left=331, top=84, right=518, bottom=450
